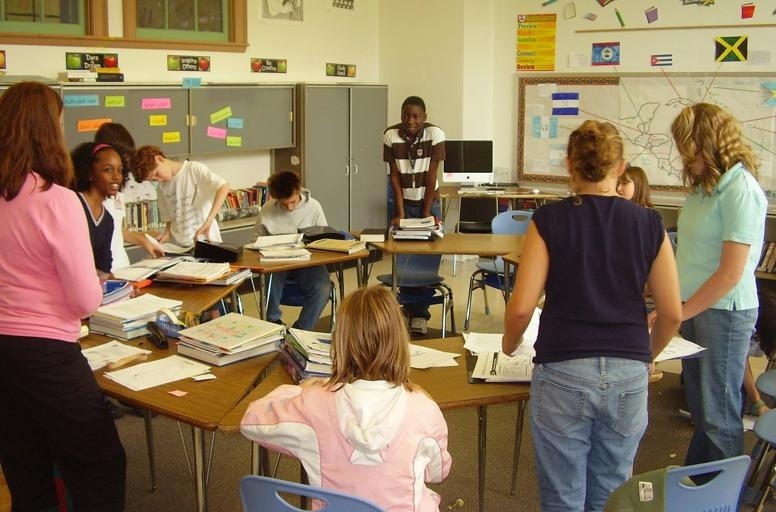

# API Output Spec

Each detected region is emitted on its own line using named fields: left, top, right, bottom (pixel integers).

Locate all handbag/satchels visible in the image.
left=193, top=239, right=243, bottom=261
left=299, top=224, right=346, bottom=242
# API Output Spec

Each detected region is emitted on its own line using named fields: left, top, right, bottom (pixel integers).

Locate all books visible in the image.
left=87, top=184, right=443, bottom=386
left=469, top=351, right=535, bottom=383
left=753, top=240, right=776, bottom=274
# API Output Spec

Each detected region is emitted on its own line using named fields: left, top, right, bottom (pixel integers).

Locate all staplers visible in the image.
left=146, top=321, right=168, bottom=348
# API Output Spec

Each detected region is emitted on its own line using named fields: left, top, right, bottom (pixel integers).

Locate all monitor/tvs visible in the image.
left=442, top=140, right=494, bottom=186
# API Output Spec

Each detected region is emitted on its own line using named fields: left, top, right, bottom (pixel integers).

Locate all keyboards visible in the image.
left=458, top=189, right=504, bottom=195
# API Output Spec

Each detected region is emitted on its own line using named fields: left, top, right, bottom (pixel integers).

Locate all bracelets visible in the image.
left=752, top=400, right=764, bottom=409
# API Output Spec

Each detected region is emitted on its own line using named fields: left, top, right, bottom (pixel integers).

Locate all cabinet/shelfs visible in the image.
left=58, top=85, right=296, bottom=158
left=270, top=83, right=389, bottom=237
left=120, top=214, right=262, bottom=293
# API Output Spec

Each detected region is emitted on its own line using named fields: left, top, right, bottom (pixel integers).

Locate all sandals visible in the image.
left=751, top=401, right=772, bottom=418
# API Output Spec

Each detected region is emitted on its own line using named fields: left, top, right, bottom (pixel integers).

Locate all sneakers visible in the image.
left=410, top=318, right=427, bottom=334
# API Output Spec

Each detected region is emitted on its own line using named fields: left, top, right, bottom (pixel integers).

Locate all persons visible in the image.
left=1, top=82, right=128, bottom=511
left=250, top=169, right=332, bottom=330
left=503, top=120, right=683, bottom=512
left=94, top=123, right=156, bottom=203
left=133, top=145, right=229, bottom=321
left=239, top=284, right=453, bottom=512
left=68, top=143, right=130, bottom=286
left=646, top=103, right=768, bottom=486
left=680, top=293, right=776, bottom=416
left=615, top=166, right=654, bottom=209
left=384, top=96, right=447, bottom=338
